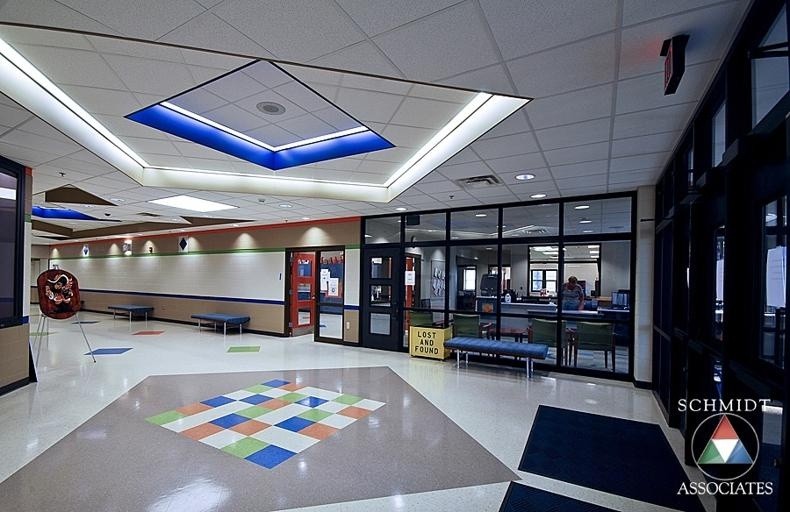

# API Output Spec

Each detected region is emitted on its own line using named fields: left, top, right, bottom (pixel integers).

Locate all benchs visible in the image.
left=108, top=304, right=153, bottom=322
left=190, top=312, right=250, bottom=337
left=442, top=335, right=549, bottom=380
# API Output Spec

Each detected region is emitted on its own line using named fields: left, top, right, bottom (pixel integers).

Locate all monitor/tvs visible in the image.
left=480, top=273, right=498, bottom=296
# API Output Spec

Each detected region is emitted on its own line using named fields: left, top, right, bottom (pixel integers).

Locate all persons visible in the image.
left=561, top=276, right=585, bottom=310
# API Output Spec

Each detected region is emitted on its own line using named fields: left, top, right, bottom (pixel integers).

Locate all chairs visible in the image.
left=407, top=310, right=617, bottom=372
